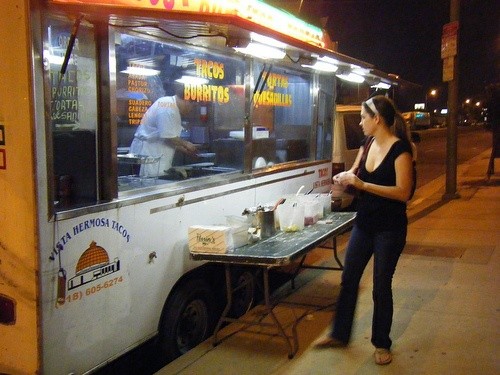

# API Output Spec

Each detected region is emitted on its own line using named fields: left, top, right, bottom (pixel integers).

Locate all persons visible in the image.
left=129, top=87, right=201, bottom=177
left=311, top=95, right=414, bottom=365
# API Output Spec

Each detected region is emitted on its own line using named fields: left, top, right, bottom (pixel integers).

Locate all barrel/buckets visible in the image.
left=277, top=203, right=305, bottom=232
left=246, top=206, right=275, bottom=238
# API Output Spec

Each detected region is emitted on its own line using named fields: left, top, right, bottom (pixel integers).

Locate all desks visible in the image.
left=188, top=212, right=358, bottom=359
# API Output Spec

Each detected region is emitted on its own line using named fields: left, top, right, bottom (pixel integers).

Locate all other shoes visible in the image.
left=374, top=348, right=392, bottom=364
left=312, top=337, right=346, bottom=348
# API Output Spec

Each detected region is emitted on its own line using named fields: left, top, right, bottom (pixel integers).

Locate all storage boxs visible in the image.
left=189, top=226, right=231, bottom=253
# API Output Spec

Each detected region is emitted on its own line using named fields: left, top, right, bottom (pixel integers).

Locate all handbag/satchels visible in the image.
left=331, top=136, right=374, bottom=201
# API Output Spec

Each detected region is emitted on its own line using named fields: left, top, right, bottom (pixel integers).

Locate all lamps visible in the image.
left=226, top=36, right=391, bottom=89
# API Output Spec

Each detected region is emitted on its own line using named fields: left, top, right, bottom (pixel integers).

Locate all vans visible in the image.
left=402, top=110, right=431, bottom=131
left=332, top=102, right=421, bottom=202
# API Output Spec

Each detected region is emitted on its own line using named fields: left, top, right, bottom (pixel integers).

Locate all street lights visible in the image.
left=471, top=102, right=481, bottom=114
left=425, top=90, right=438, bottom=111
left=461, top=99, right=471, bottom=128
